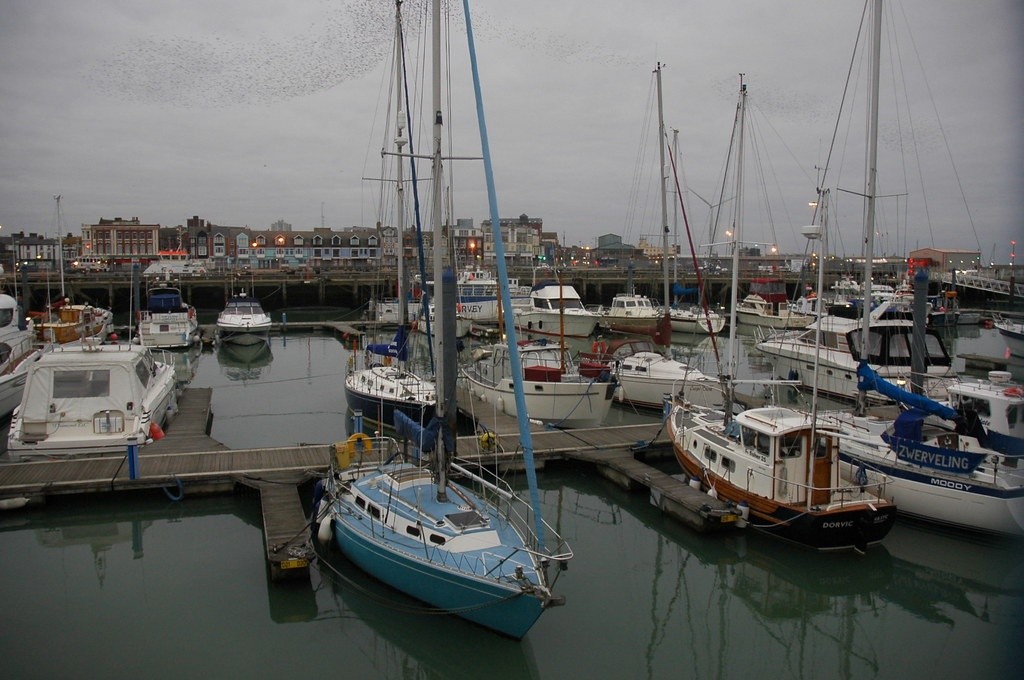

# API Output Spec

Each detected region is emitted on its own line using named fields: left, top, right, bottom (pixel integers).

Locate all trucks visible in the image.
left=75, top=257, right=110, bottom=272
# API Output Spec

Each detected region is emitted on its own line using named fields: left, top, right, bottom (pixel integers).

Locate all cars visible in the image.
left=963, top=270, right=985, bottom=285
left=951, top=270, right=966, bottom=282
left=537, top=263, right=549, bottom=268
left=990, top=314, right=1024, bottom=358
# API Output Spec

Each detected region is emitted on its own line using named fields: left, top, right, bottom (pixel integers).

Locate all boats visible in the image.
left=424, top=266, right=532, bottom=321
left=596, top=294, right=662, bottom=335
left=216, top=288, right=272, bottom=345
left=0, top=266, right=46, bottom=416
left=788, top=274, right=915, bottom=317
left=755, top=301, right=966, bottom=406
left=736, top=278, right=815, bottom=328
left=577, top=338, right=723, bottom=409
left=7, top=344, right=179, bottom=462
left=461, top=269, right=622, bottom=428
left=138, top=266, right=198, bottom=349
left=516, top=284, right=605, bottom=337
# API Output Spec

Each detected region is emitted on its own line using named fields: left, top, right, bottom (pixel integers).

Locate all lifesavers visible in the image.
left=346, top=432, right=373, bottom=460
left=480, top=430, right=501, bottom=451
left=592, top=339, right=607, bottom=354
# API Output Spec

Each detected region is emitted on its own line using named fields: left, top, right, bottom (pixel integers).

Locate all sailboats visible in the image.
left=311, top=1, right=573, bottom=641
left=665, top=85, right=898, bottom=551
left=26, top=194, right=113, bottom=346
left=344, top=1, right=437, bottom=433
left=671, top=129, right=726, bottom=333
left=804, top=1, right=1024, bottom=538
